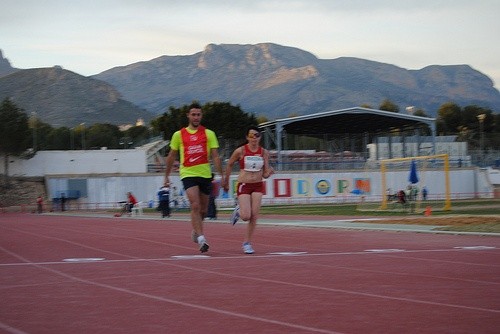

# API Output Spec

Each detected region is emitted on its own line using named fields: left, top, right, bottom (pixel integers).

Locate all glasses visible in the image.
left=248, top=132, right=261, bottom=138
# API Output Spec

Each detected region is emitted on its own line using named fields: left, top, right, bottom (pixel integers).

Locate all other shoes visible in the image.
left=192, top=228, right=199, bottom=244
left=199, top=239, right=209, bottom=252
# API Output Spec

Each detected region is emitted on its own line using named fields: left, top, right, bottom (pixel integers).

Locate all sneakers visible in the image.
left=242, top=241, right=254, bottom=254
left=232, top=204, right=240, bottom=225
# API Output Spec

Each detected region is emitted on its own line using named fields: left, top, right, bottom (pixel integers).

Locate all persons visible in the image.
left=386, top=184, right=428, bottom=204
left=224, top=127, right=277, bottom=253
left=123, top=192, right=136, bottom=214
left=164, top=103, right=225, bottom=253
left=158, top=180, right=171, bottom=218
left=37, top=195, right=43, bottom=213
left=60, top=195, right=66, bottom=211
left=173, top=186, right=179, bottom=211
left=205, top=173, right=219, bottom=220
